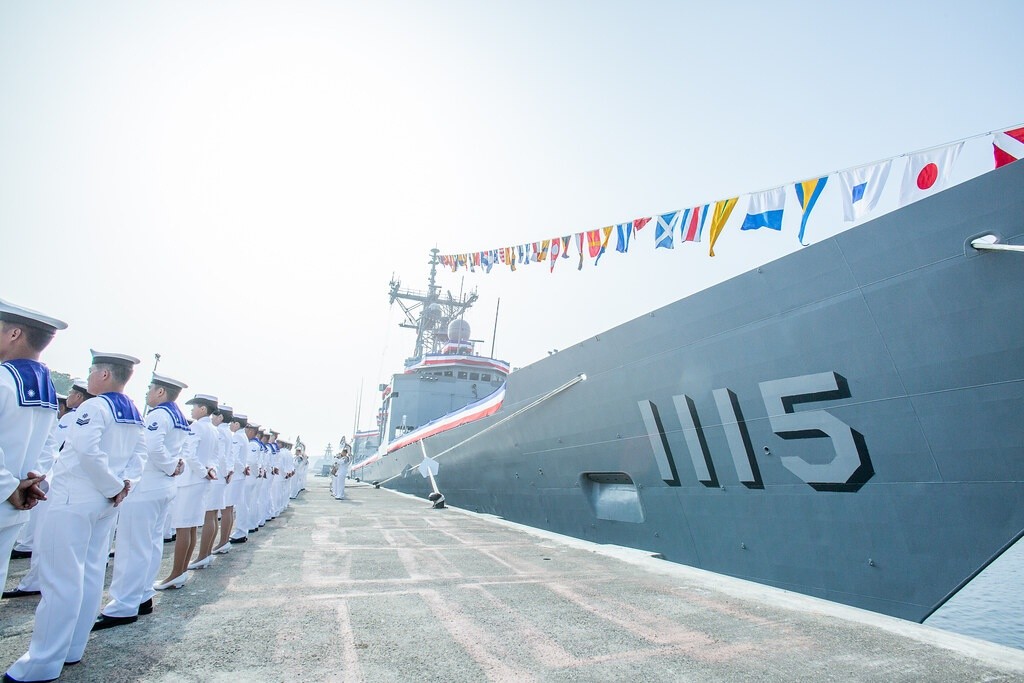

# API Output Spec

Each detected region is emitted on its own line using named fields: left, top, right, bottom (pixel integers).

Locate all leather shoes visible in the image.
left=0, top=516, right=277, bottom=683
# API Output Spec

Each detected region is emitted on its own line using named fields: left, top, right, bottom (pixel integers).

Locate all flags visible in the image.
left=899, top=140, right=965, bottom=203
left=740, top=187, right=787, bottom=230
left=992, top=127, right=1024, bottom=168
left=436, top=196, right=738, bottom=272
left=840, top=160, right=892, bottom=221
left=795, top=177, right=831, bottom=247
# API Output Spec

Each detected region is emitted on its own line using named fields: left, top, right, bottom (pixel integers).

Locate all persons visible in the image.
left=93, top=370, right=188, bottom=630
left=152, top=394, right=309, bottom=589
left=0, top=349, right=146, bottom=683
left=2, top=380, right=97, bottom=597
left=329, top=449, right=349, bottom=499
left=0, top=294, right=69, bottom=602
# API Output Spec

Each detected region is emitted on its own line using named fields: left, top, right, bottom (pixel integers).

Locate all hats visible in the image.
left=185, top=394, right=219, bottom=409
left=90, top=349, right=140, bottom=369
left=245, top=423, right=261, bottom=428
left=232, top=414, right=248, bottom=420
left=151, top=372, right=189, bottom=392
left=71, top=380, right=97, bottom=398
left=56, top=393, right=69, bottom=403
left=270, top=431, right=280, bottom=438
left=263, top=433, right=272, bottom=436
left=257, top=428, right=266, bottom=433
left=0, top=299, right=68, bottom=334
left=217, top=405, right=233, bottom=412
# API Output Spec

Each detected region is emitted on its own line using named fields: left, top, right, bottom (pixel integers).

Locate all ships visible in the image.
left=346, top=158, right=1024, bottom=625
